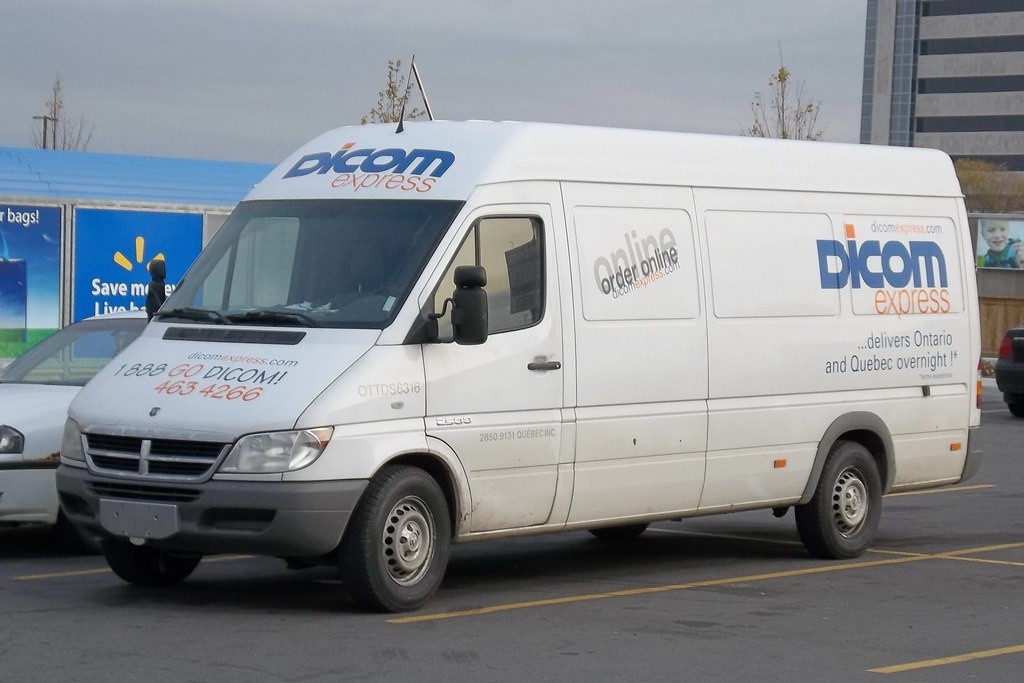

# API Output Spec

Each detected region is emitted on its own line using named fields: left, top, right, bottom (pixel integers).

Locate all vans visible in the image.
left=56, top=120, right=983, bottom=614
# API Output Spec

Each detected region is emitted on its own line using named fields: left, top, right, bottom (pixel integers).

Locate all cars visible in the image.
left=995, top=322, right=1024, bottom=417
left=0, top=310, right=148, bottom=555
